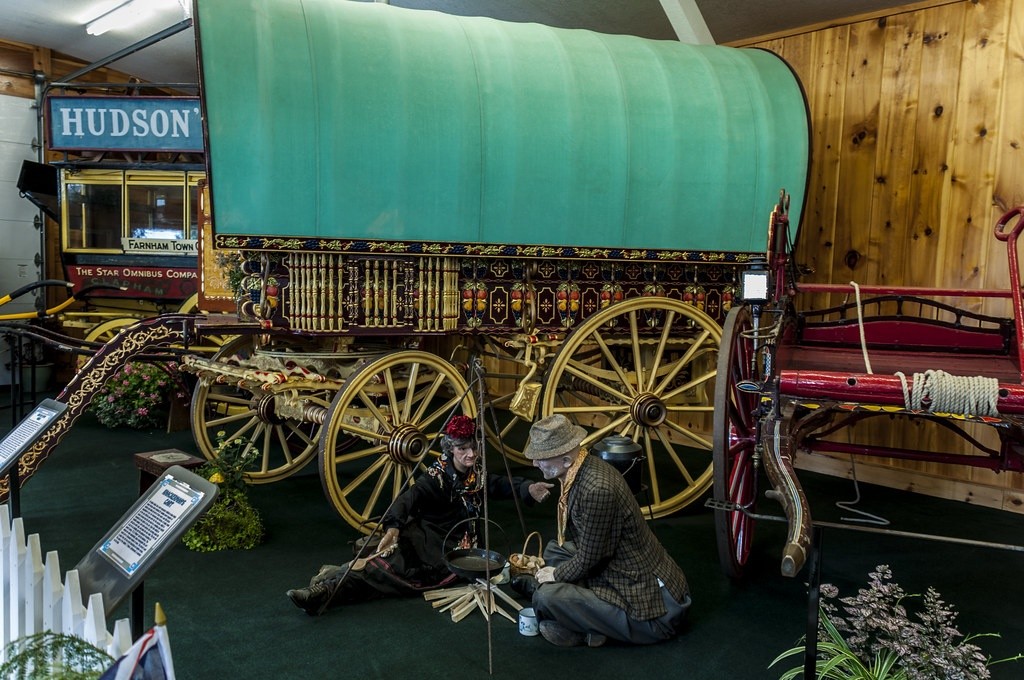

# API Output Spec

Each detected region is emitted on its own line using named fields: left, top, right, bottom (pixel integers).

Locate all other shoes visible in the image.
left=538, top=621, right=606, bottom=647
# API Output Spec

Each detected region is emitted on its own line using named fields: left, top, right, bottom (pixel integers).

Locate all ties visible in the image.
left=557, top=449, right=589, bottom=548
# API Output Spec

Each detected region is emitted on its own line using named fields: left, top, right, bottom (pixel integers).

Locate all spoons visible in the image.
left=348, top=543, right=398, bottom=571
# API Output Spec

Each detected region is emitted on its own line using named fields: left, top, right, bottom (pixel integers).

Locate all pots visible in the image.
left=441, top=518, right=510, bottom=578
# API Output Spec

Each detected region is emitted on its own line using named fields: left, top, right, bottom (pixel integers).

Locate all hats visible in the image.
left=525, top=413, right=587, bottom=460
left=446, top=415, right=476, bottom=440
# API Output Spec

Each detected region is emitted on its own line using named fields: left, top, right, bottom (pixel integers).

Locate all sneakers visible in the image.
left=286, top=582, right=330, bottom=617
left=310, top=565, right=341, bottom=589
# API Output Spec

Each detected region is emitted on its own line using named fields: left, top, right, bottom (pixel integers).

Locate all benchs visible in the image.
left=771, top=284, right=1024, bottom=422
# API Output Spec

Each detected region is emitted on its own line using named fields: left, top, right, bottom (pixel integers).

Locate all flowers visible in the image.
left=93, top=361, right=184, bottom=428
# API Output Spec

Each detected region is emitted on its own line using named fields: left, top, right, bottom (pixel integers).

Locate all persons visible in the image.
left=495, top=414, right=692, bottom=645
left=284, top=415, right=554, bottom=618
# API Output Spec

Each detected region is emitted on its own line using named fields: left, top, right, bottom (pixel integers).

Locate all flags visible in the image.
left=99, top=625, right=176, bottom=680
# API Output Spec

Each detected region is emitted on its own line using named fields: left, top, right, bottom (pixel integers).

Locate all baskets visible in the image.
left=508, top=530, right=545, bottom=578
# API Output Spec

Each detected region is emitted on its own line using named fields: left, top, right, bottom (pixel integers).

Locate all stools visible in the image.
left=135, top=448, right=206, bottom=497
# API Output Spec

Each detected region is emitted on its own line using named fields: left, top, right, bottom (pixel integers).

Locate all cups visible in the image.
left=518, top=607, right=540, bottom=636
left=496, top=562, right=510, bottom=584
